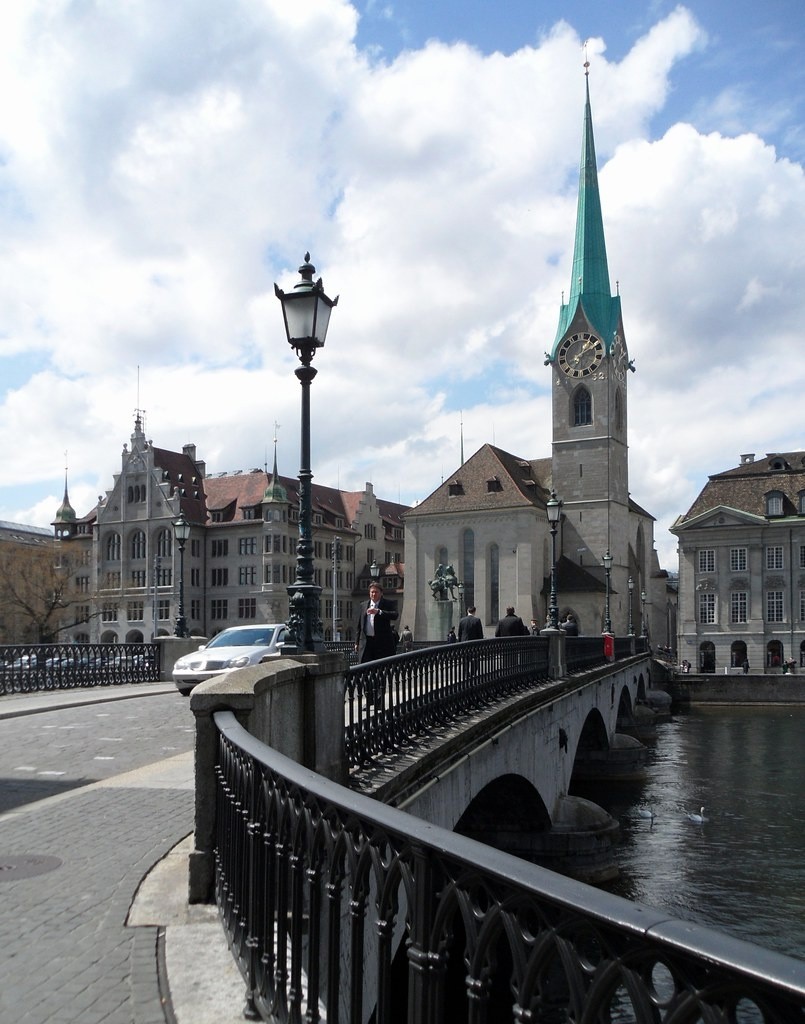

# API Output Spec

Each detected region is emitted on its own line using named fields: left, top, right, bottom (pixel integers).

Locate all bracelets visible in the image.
left=378, top=609, right=380, bottom=614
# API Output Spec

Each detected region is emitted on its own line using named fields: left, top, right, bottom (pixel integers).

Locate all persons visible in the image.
left=656, top=644, right=677, bottom=660
left=355, top=581, right=400, bottom=710
left=447, top=626, right=456, bottom=643
left=457, top=606, right=483, bottom=677
left=390, top=624, right=414, bottom=654
left=495, top=605, right=578, bottom=667
left=681, top=660, right=690, bottom=672
left=434, top=564, right=448, bottom=590
left=741, top=659, right=750, bottom=674
left=773, top=654, right=798, bottom=674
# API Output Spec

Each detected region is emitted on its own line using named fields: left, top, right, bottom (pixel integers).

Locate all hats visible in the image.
left=531, top=618, right=537, bottom=621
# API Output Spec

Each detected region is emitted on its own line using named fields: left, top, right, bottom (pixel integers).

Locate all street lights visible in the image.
left=369, top=559, right=381, bottom=583
left=640, top=589, right=647, bottom=635
left=273, top=248, right=340, bottom=770
left=627, top=575, right=635, bottom=634
left=603, top=548, right=614, bottom=634
left=544, top=489, right=563, bottom=631
left=172, top=508, right=191, bottom=637
left=458, top=583, right=464, bottom=618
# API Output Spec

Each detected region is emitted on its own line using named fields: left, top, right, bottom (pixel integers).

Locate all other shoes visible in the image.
left=362, top=701, right=374, bottom=711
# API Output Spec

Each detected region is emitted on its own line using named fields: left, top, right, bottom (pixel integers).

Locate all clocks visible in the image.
left=610, top=334, right=626, bottom=382
left=557, top=331, right=603, bottom=379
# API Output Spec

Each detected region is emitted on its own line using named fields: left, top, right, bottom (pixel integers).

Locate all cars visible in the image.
left=172, top=623, right=287, bottom=697
left=6, top=653, right=143, bottom=672
